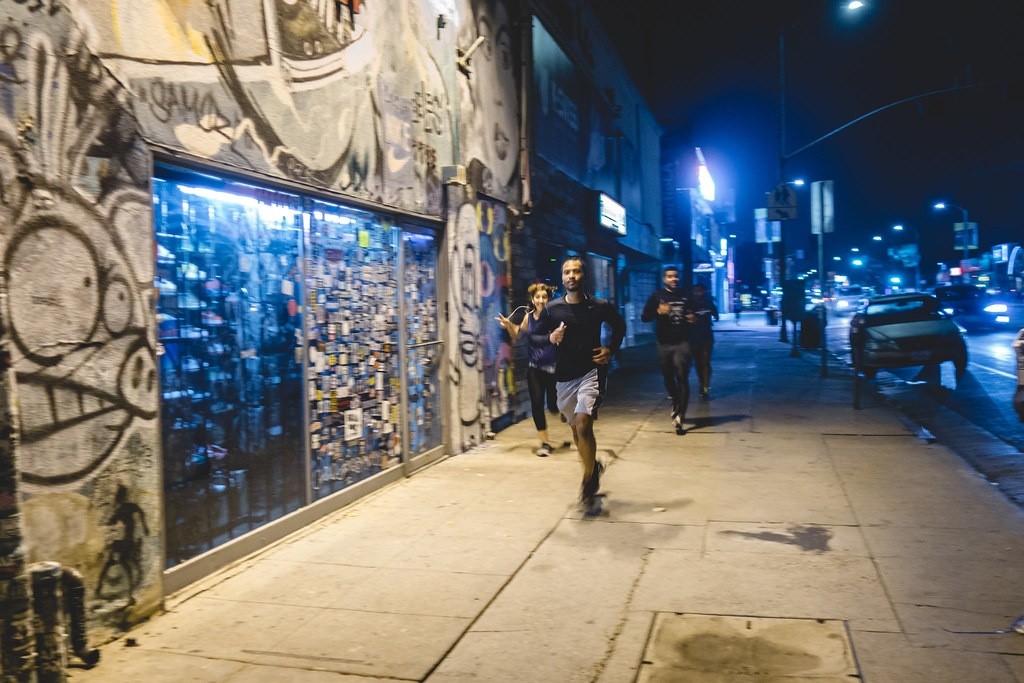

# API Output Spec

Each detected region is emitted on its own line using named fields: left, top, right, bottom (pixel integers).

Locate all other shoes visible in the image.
left=537, top=441, right=552, bottom=457
left=670, top=397, right=683, bottom=434
left=698, top=383, right=710, bottom=403
left=576, top=459, right=606, bottom=517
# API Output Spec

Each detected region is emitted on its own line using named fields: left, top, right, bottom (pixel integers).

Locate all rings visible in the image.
left=605, top=361, right=609, bottom=365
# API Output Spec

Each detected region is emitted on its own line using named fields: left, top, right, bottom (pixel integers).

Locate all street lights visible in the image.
left=778, top=74, right=1011, bottom=341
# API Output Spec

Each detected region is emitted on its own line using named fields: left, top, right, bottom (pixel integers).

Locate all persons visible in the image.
left=529, top=255, right=626, bottom=514
left=689, top=283, right=719, bottom=399
left=642, top=267, right=698, bottom=436
left=732, top=293, right=743, bottom=326
left=494, top=283, right=569, bottom=457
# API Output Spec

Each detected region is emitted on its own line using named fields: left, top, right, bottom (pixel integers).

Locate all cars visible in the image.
left=934, top=284, right=1010, bottom=332
left=850, top=292, right=969, bottom=378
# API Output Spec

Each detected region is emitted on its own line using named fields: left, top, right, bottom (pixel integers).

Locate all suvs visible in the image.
left=832, top=284, right=864, bottom=315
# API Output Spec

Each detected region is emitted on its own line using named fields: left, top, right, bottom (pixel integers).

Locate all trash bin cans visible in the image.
left=763, top=306, right=781, bottom=325
left=801, top=302, right=826, bottom=348
